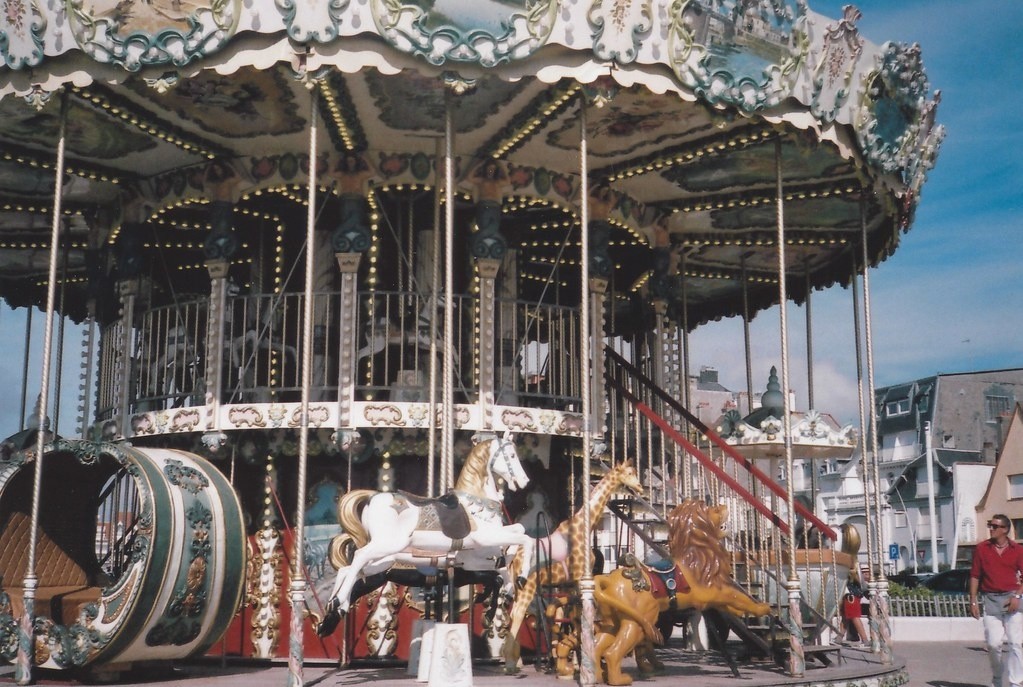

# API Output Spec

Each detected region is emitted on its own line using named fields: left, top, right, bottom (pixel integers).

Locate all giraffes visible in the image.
left=503, top=455, right=644, bottom=676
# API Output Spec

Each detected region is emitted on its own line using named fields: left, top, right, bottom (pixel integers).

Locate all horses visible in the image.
left=316, top=431, right=533, bottom=639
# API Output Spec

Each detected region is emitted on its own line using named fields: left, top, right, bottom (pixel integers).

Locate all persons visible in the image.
left=834, top=566, right=871, bottom=647
left=970, top=515, right=1023, bottom=687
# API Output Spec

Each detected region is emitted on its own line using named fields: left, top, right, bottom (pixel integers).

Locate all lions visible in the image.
left=590, top=496, right=771, bottom=686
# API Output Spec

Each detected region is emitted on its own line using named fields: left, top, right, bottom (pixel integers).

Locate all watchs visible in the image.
left=1013, top=594, right=1022, bottom=599
left=970, top=603, right=977, bottom=606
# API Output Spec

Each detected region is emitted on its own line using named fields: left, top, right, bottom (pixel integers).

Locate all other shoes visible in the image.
left=992, top=676, right=1003, bottom=687
left=858, top=644, right=871, bottom=649
left=847, top=634, right=861, bottom=641
left=1011, top=683, right=1023, bottom=687
left=829, top=640, right=842, bottom=648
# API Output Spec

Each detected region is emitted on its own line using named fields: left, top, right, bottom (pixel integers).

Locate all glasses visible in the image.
left=986, top=520, right=1006, bottom=530
